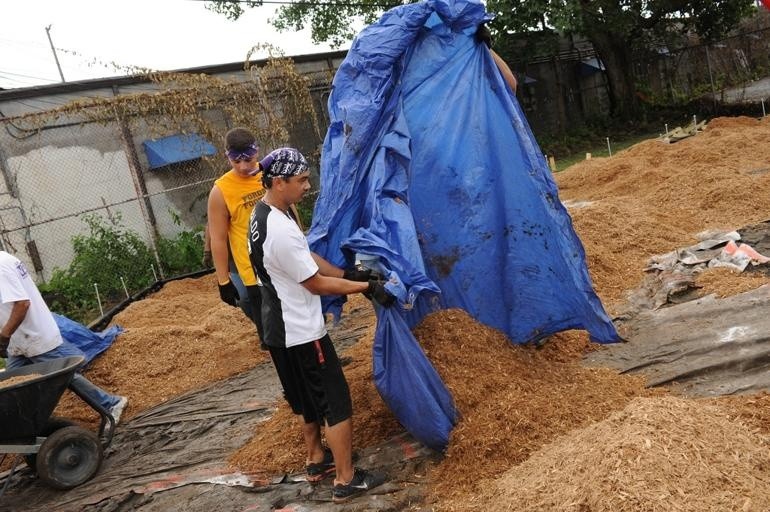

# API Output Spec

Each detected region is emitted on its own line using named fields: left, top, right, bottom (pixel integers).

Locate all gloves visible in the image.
left=344, top=271, right=378, bottom=300
left=218, top=278, right=241, bottom=308
left=202, top=253, right=212, bottom=267
left=369, top=280, right=397, bottom=308
left=474, top=24, right=493, bottom=48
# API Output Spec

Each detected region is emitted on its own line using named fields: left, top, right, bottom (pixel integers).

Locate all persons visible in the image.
left=202, top=173, right=256, bottom=325
left=0, top=250, right=127, bottom=431
left=248, top=147, right=400, bottom=505
left=480, top=24, right=520, bottom=96
left=207, top=127, right=355, bottom=367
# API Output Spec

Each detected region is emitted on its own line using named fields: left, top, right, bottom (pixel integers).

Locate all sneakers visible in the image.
left=305, top=448, right=358, bottom=481
left=104, top=396, right=128, bottom=430
left=332, top=466, right=387, bottom=503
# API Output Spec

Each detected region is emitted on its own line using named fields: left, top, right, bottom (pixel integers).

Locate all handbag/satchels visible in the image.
left=0, top=334, right=9, bottom=358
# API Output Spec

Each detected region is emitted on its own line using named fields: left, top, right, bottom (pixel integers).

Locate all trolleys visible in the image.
left=0, top=354, right=116, bottom=493
